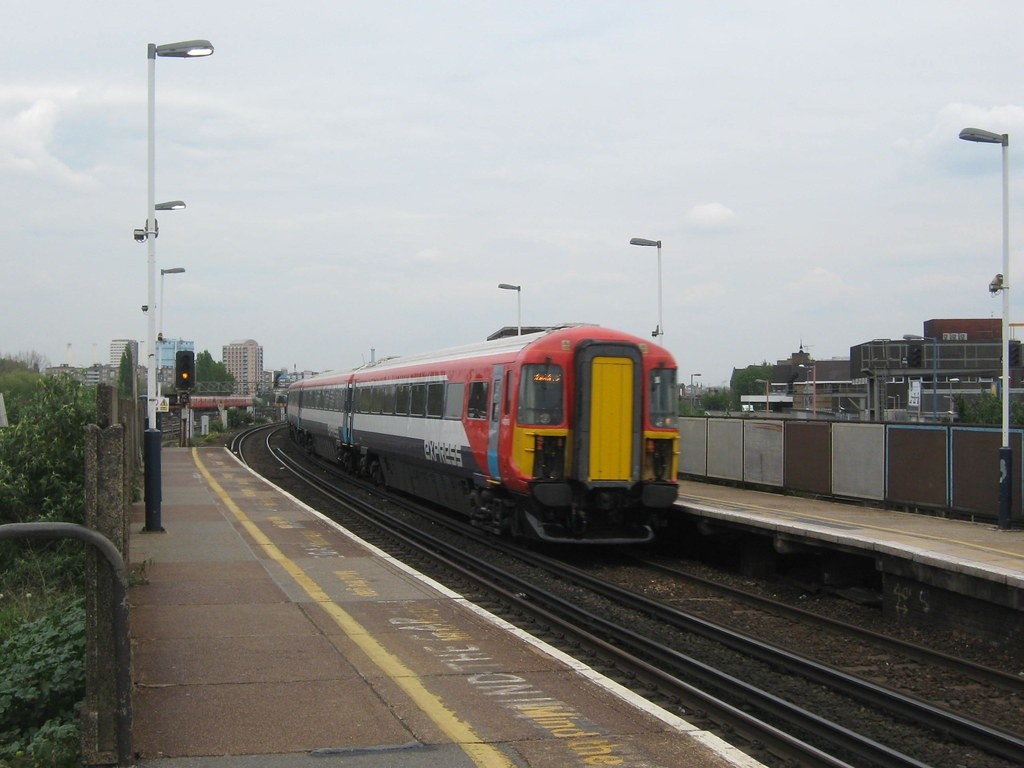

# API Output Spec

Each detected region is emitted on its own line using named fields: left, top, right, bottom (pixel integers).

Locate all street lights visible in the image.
left=901, top=334, right=939, bottom=426
left=157, top=268, right=185, bottom=402
left=799, top=364, right=817, bottom=417
left=497, top=283, right=523, bottom=339
left=690, top=374, right=702, bottom=417
left=629, top=237, right=664, bottom=350
left=957, top=127, right=1015, bottom=532
left=136, top=40, right=215, bottom=535
left=948, top=378, right=960, bottom=427
left=755, top=379, right=770, bottom=416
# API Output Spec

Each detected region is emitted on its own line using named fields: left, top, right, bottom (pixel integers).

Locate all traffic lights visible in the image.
left=175, top=350, right=196, bottom=388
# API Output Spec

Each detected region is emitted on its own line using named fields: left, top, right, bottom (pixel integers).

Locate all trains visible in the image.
left=286, top=322, right=685, bottom=565
